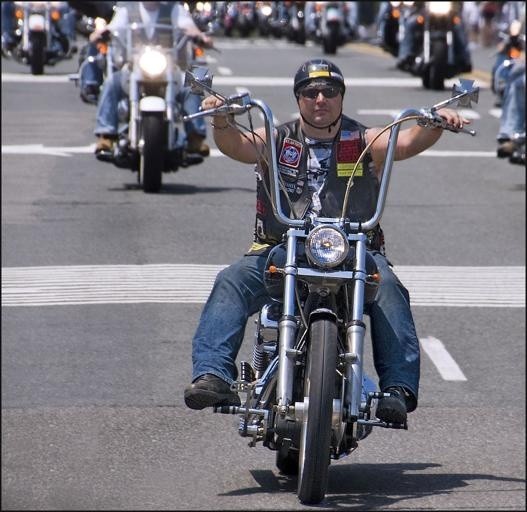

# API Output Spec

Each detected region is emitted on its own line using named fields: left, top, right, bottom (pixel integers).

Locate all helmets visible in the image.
left=293, top=59, right=344, bottom=97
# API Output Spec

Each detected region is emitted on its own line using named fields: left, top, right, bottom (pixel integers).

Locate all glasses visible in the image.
left=297, top=86, right=343, bottom=97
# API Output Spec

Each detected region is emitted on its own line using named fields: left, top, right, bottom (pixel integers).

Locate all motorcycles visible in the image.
left=373, top=2, right=527, bottom=166
left=0, top=1, right=78, bottom=76
left=86, top=1, right=221, bottom=192
left=184, top=64, right=479, bottom=502
left=190, top=2, right=356, bottom=53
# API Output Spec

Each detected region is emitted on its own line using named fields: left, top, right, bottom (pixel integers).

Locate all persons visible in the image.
left=95, top=1, right=210, bottom=157
left=356, top=2, right=472, bottom=76
left=185, top=60, right=471, bottom=425
left=1, top=0, right=116, bottom=97
left=463, top=2, right=525, bottom=160
left=189, top=1, right=359, bottom=46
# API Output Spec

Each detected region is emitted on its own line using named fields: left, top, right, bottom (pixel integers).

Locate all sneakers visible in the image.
left=186, top=137, right=208, bottom=157
left=184, top=374, right=241, bottom=409
left=375, top=387, right=408, bottom=428
left=95, top=138, right=113, bottom=153
left=497, top=140, right=522, bottom=156
